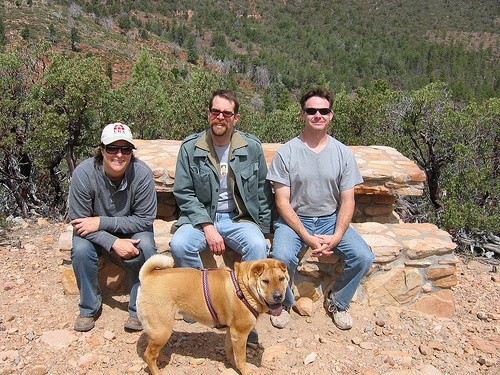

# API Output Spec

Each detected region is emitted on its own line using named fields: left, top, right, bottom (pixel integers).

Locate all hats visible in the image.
left=101, top=122, right=137, bottom=149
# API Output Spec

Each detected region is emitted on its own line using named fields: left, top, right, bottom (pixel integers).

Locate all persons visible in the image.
left=266, top=86, right=374, bottom=330
left=69, top=123, right=157, bottom=330
left=171, top=89, right=273, bottom=343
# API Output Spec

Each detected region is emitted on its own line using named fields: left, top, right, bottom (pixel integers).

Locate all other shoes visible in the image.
left=183, top=315, right=197, bottom=324
left=246, top=327, right=259, bottom=343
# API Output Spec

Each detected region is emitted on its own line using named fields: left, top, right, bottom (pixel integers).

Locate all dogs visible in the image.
left=135, top=252, right=291, bottom=375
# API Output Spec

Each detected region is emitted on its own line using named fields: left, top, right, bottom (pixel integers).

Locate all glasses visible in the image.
left=210, top=108, right=234, bottom=118
left=305, top=108, right=332, bottom=115
left=101, top=144, right=133, bottom=155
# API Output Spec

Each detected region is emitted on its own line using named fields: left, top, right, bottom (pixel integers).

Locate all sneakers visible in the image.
left=325, top=288, right=353, bottom=329
left=124, top=316, right=144, bottom=330
left=270, top=304, right=291, bottom=327
left=74, top=303, right=102, bottom=330
